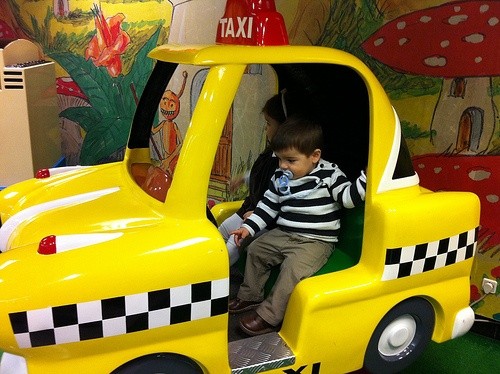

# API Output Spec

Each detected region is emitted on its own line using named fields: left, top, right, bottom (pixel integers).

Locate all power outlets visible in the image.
left=482, top=277, right=498, bottom=296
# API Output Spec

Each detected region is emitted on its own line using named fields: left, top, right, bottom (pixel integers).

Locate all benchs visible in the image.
left=232, top=201, right=364, bottom=299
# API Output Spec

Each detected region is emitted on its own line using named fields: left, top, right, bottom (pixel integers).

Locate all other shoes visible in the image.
left=228, top=297, right=262, bottom=313
left=239, top=310, right=276, bottom=335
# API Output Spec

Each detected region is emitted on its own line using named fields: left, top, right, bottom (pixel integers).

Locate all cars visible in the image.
left=1, top=0, right=483, bottom=374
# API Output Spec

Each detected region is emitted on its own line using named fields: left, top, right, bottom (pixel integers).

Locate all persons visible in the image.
left=228, top=118, right=368, bottom=336
left=219, top=89, right=333, bottom=268
